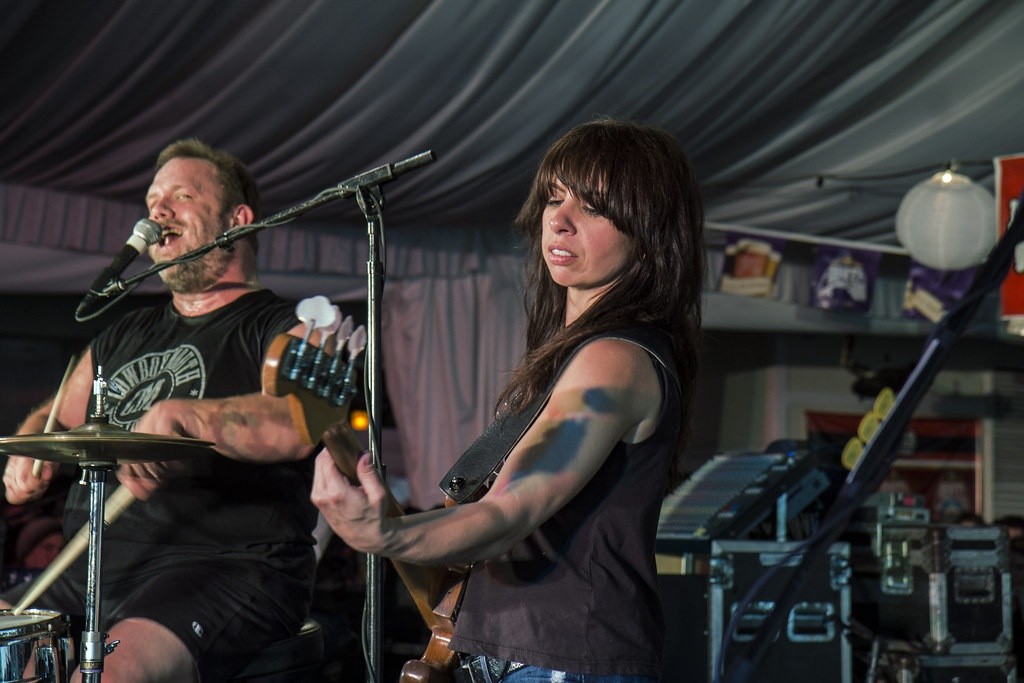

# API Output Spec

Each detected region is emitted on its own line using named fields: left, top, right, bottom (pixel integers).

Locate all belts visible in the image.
left=452, top=655, right=528, bottom=683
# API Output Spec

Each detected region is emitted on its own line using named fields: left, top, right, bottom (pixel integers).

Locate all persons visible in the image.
left=306, top=115, right=710, bottom=683
left=3, top=136, right=342, bottom=683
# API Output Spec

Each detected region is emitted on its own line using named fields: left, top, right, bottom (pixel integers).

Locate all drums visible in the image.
left=0, top=610, right=77, bottom=683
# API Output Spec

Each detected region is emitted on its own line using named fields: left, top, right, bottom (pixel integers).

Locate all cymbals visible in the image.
left=0, top=423, right=217, bottom=465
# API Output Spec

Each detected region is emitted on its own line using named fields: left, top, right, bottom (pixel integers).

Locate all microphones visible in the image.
left=76, top=219, right=162, bottom=318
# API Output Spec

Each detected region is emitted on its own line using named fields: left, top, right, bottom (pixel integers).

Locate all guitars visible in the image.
left=262, top=295, right=489, bottom=680
